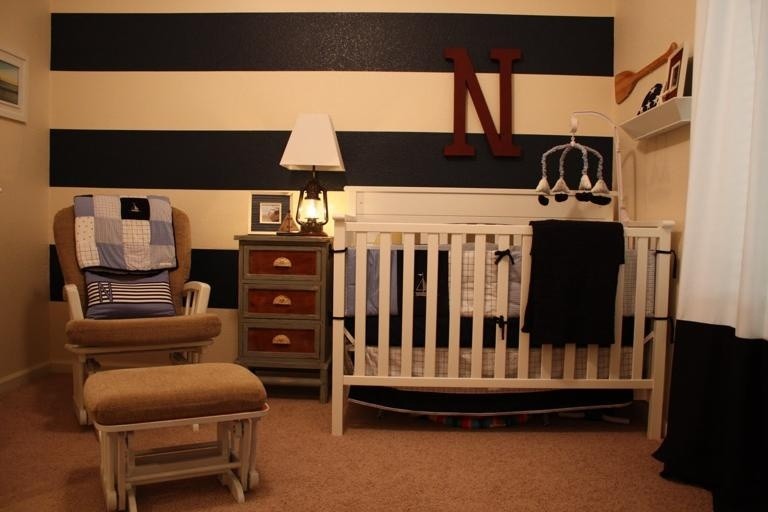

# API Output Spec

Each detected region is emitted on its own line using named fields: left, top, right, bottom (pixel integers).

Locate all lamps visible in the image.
left=280, top=110, right=346, bottom=236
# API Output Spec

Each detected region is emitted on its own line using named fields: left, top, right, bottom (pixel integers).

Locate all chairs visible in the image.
left=52, top=194, right=222, bottom=425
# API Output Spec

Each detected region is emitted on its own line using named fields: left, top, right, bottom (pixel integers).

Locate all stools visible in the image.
left=83, top=362, right=271, bottom=512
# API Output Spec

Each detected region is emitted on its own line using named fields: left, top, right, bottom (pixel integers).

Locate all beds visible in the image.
left=330, top=213, right=675, bottom=441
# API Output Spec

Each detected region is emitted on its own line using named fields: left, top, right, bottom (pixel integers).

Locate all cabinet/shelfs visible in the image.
left=233, top=232, right=331, bottom=405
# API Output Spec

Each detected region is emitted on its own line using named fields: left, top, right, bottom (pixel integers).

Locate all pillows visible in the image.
left=82, top=270, right=177, bottom=319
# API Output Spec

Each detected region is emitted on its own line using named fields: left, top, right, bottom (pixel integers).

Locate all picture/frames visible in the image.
left=659, top=40, right=690, bottom=104
left=1, top=47, right=29, bottom=126
left=250, top=189, right=294, bottom=236
